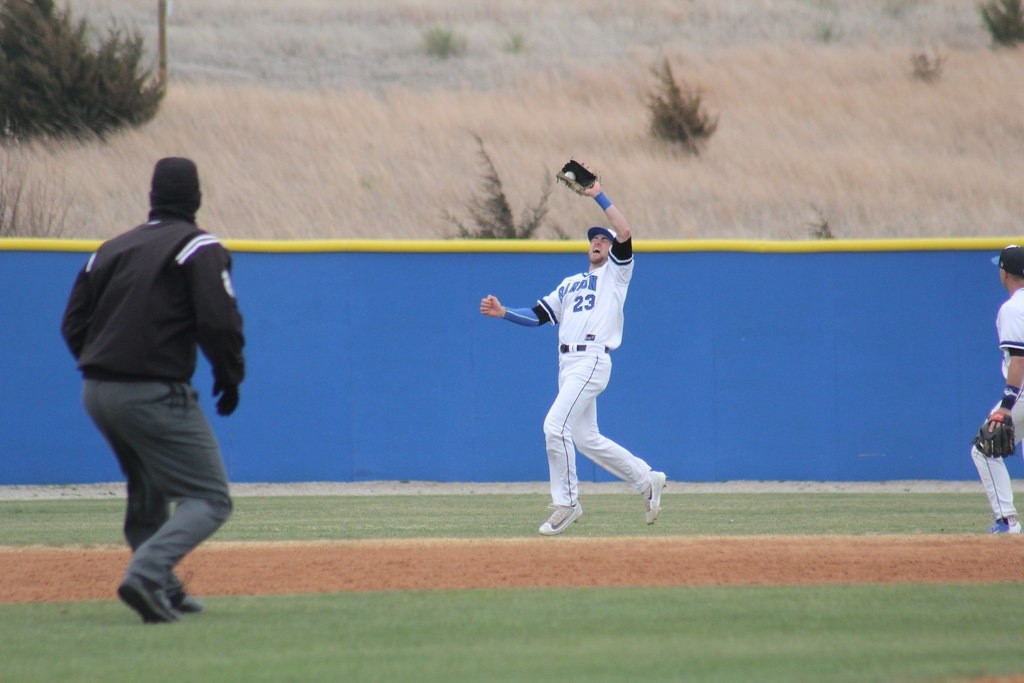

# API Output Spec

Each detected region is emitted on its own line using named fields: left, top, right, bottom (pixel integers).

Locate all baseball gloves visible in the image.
left=969, top=411, right=1016, bottom=458
left=555, top=155, right=602, bottom=197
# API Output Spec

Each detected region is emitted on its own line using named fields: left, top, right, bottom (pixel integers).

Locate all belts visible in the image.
left=560, top=344, right=609, bottom=354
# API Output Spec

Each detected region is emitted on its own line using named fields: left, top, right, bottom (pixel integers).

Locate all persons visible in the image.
left=481, top=160, right=666, bottom=536
left=60, top=157, right=246, bottom=623
left=971, top=245, right=1024, bottom=535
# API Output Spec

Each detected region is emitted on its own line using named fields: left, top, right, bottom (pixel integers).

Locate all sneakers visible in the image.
left=640, top=471, right=670, bottom=526
left=538, top=500, right=583, bottom=536
left=988, top=517, right=1022, bottom=534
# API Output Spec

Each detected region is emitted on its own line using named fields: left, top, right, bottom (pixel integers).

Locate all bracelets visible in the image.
left=594, top=191, right=612, bottom=211
left=1001, top=384, right=1022, bottom=410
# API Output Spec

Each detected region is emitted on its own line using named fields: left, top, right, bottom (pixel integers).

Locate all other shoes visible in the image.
left=170, top=571, right=203, bottom=612
left=117, top=571, right=180, bottom=626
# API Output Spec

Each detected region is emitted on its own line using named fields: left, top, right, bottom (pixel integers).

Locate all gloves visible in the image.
left=212, top=379, right=240, bottom=417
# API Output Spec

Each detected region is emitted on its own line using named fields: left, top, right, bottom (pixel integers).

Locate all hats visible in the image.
left=587, top=226, right=616, bottom=244
left=990, top=245, right=1024, bottom=277
left=149, top=158, right=201, bottom=212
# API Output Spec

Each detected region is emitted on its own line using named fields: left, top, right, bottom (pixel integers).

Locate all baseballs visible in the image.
left=564, top=171, right=576, bottom=181
